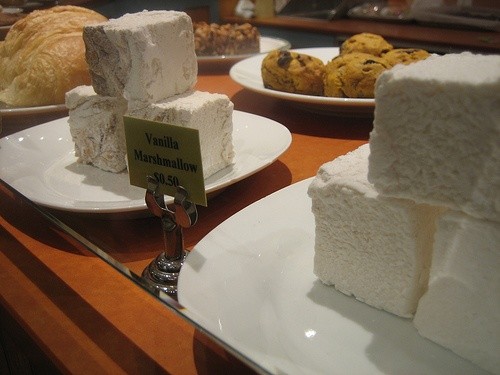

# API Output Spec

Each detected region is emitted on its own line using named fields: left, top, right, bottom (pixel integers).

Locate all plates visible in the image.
left=346, top=6, right=416, bottom=20
left=1, top=7, right=24, bottom=14
left=177, top=177, right=498, bottom=375
left=0, top=110, right=292, bottom=220
left=0, top=25, right=11, bottom=29
left=229, top=47, right=441, bottom=117
left=0, top=103, right=69, bottom=120
left=198, top=35, right=291, bottom=69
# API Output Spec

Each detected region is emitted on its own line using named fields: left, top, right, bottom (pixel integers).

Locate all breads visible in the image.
left=311, top=53, right=500, bottom=375
left=2, top=5, right=110, bottom=107
left=191, top=20, right=260, bottom=56
left=64, top=10, right=234, bottom=181
left=260, top=33, right=433, bottom=98
